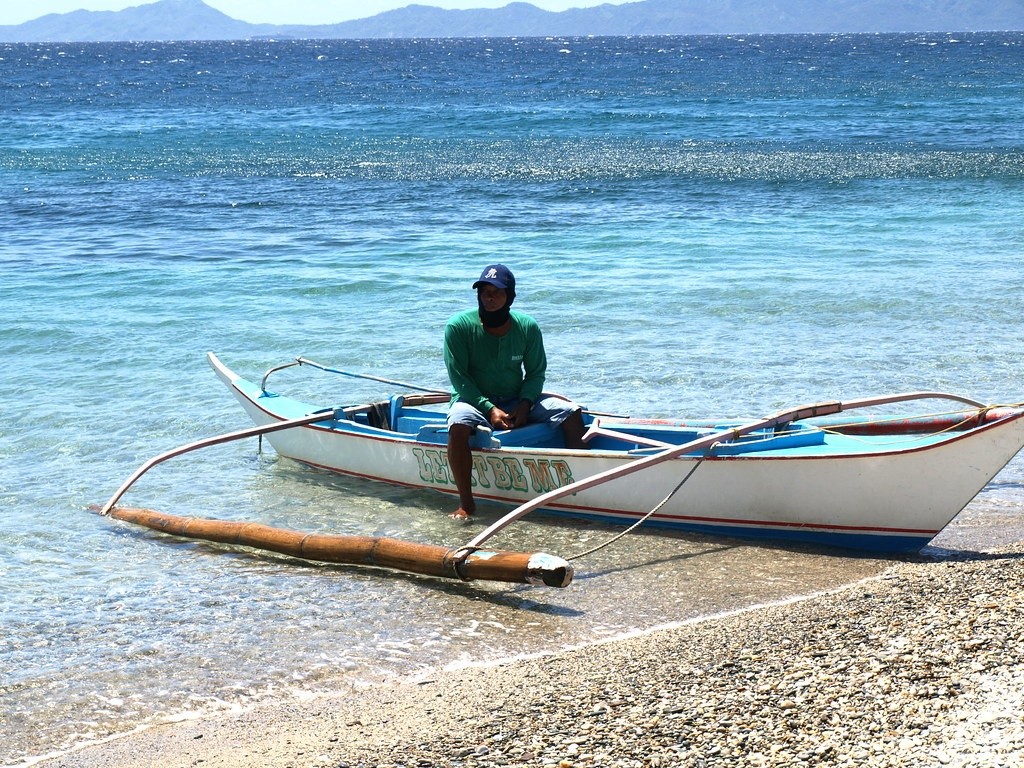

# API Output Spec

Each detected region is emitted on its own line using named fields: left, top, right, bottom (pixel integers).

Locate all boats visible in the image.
left=202, top=349, right=1024, bottom=560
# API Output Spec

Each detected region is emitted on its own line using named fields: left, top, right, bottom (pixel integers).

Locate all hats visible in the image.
left=472, top=263, right=515, bottom=289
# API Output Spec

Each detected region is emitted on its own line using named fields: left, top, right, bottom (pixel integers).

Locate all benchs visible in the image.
left=412, top=404, right=589, bottom=448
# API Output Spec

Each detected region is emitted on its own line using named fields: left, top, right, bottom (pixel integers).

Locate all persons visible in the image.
left=444, top=264, right=585, bottom=518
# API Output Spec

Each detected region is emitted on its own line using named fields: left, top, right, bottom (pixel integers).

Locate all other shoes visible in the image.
left=452, top=499, right=476, bottom=524
